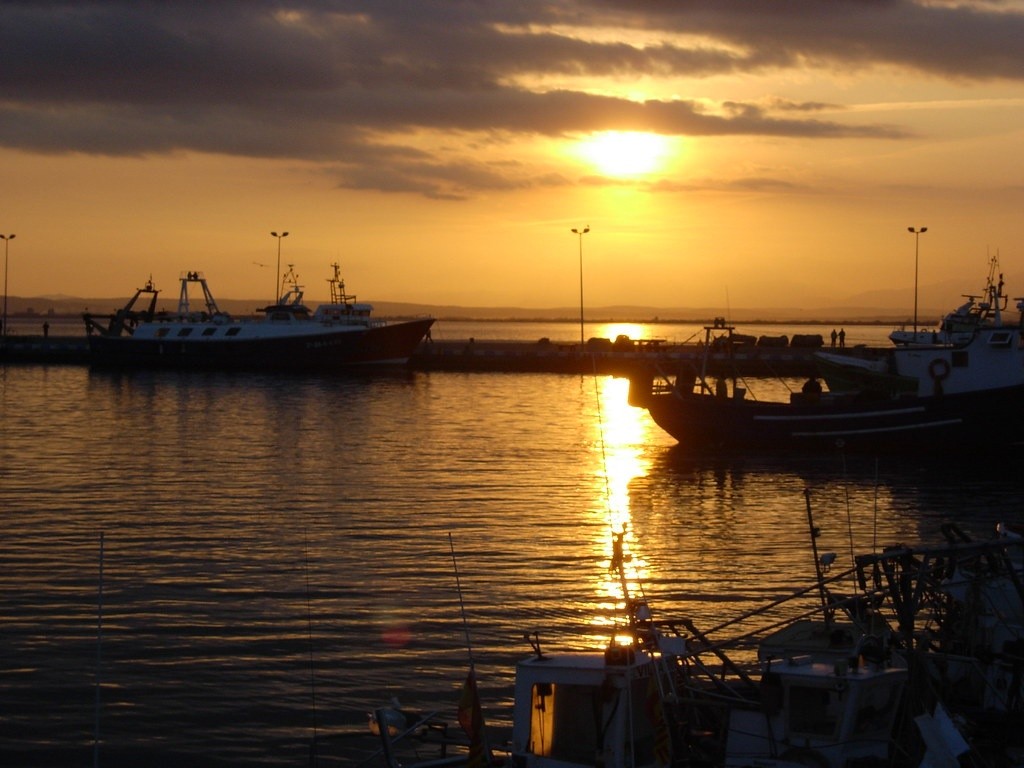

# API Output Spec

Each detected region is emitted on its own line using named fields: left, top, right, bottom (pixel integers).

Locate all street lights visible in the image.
left=271, top=231, right=289, bottom=306
left=0, top=234, right=17, bottom=335
left=908, top=226, right=929, bottom=334
left=571, top=228, right=592, bottom=346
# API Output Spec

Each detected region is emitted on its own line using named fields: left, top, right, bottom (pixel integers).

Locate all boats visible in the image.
left=629, top=246, right=1024, bottom=462
left=302, top=481, right=1024, bottom=768
left=82, top=262, right=438, bottom=375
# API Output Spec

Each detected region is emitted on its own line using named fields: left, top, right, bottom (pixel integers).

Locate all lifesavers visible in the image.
left=928, top=358, right=951, bottom=381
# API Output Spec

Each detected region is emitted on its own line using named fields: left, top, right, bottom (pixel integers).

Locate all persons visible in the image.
left=830, top=328, right=846, bottom=348
left=423, top=328, right=434, bottom=344
left=41, top=321, right=50, bottom=337
